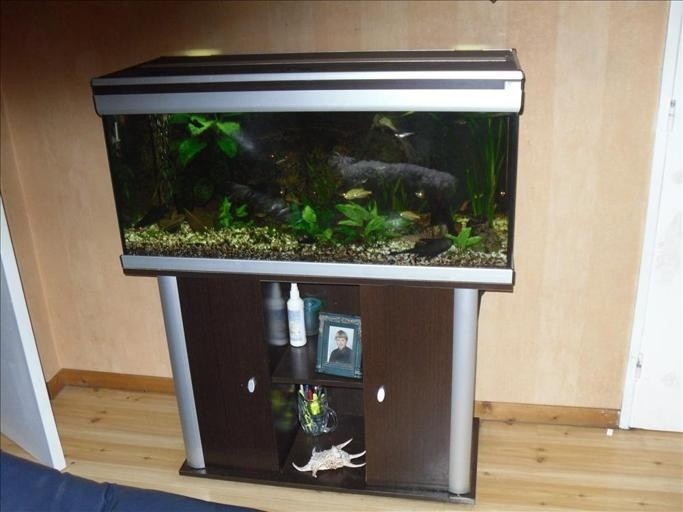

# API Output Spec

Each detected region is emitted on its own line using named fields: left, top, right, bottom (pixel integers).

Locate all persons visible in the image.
left=330, top=330, right=353, bottom=366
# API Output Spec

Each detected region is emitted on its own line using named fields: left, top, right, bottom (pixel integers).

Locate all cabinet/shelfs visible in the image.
left=157, top=274, right=479, bottom=508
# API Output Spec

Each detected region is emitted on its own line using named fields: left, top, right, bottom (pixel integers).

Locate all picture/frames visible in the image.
left=312, top=310, right=362, bottom=380
